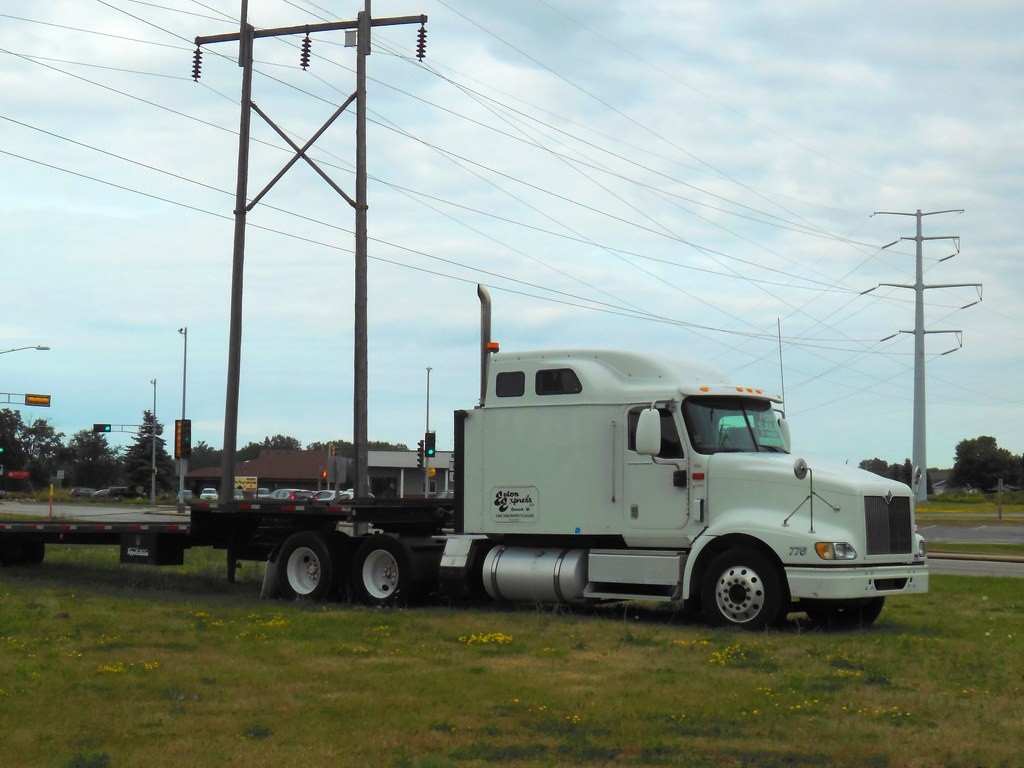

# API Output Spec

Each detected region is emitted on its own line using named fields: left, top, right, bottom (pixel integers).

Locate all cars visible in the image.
left=234, top=489, right=244, bottom=500
left=300, top=489, right=354, bottom=501
left=69, top=487, right=98, bottom=496
left=92, top=486, right=145, bottom=501
left=176, top=490, right=193, bottom=500
left=271, top=489, right=307, bottom=502
left=200, top=488, right=218, bottom=500
left=253, top=488, right=272, bottom=499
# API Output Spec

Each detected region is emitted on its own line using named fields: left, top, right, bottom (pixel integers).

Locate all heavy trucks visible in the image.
left=0, top=282, right=928, bottom=633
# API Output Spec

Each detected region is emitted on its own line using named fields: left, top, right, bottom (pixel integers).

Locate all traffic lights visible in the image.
left=181, top=420, right=191, bottom=459
left=416, top=440, right=424, bottom=471
left=322, top=471, right=328, bottom=477
left=93, top=425, right=111, bottom=432
left=425, top=433, right=435, bottom=457
